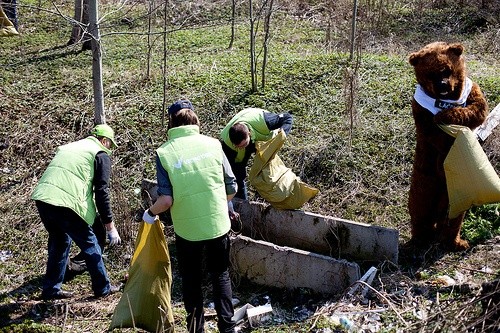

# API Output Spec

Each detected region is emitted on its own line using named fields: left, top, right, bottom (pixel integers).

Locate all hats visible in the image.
left=168, top=100, right=194, bottom=115
left=91, top=125, right=118, bottom=148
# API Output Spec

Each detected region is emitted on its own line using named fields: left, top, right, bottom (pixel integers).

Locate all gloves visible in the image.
left=107, top=226, right=121, bottom=246
left=142, top=209, right=157, bottom=224
left=228, top=201, right=234, bottom=214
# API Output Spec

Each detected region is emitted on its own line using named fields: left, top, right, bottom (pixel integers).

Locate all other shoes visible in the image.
left=110, top=282, right=123, bottom=294
left=56, top=289, right=75, bottom=298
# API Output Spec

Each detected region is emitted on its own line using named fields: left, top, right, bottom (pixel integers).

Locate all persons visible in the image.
left=31, top=124, right=121, bottom=298
left=142, top=101, right=239, bottom=333
left=221, top=108, right=291, bottom=213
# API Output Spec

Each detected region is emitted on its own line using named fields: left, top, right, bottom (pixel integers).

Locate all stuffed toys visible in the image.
left=406, top=42, right=487, bottom=262
